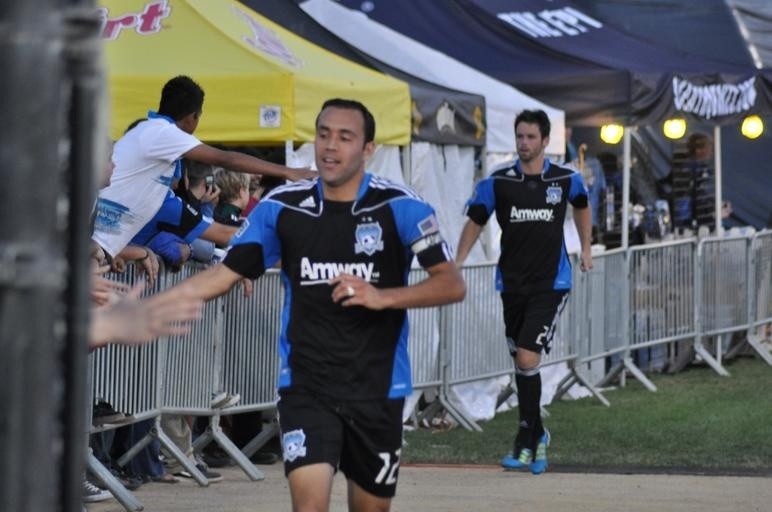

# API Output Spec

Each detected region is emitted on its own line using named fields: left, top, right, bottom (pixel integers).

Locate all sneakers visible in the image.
left=81, top=448, right=280, bottom=503
left=500, top=427, right=551, bottom=474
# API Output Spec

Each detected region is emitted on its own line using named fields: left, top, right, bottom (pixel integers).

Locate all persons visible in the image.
left=90, top=74, right=319, bottom=258
left=185, top=97, right=467, bottom=511
left=86, top=153, right=288, bottom=500
left=457, top=109, right=593, bottom=473
left=568, top=131, right=733, bottom=376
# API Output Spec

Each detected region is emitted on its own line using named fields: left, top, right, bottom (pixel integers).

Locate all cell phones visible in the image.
left=206, top=176, right=215, bottom=192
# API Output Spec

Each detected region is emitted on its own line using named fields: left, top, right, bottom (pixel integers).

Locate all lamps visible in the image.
left=741, top=114, right=763, bottom=139
left=601, top=124, right=623, bottom=144
left=663, top=118, right=685, bottom=139
